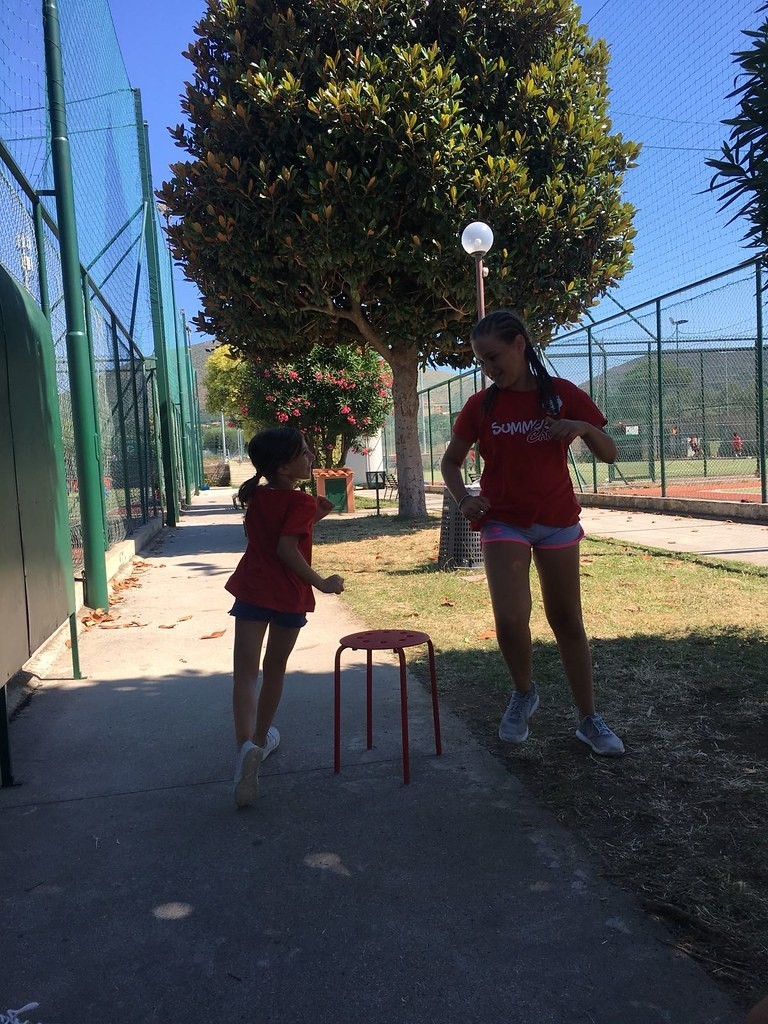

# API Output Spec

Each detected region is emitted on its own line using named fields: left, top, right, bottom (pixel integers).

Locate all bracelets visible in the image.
left=458, top=494, right=473, bottom=512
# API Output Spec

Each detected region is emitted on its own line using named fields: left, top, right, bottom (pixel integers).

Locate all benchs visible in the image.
left=232, top=493, right=243, bottom=510
left=380, top=472, right=399, bottom=501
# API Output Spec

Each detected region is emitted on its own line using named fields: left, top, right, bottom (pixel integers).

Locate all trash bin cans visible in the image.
left=439, top=483, right=486, bottom=570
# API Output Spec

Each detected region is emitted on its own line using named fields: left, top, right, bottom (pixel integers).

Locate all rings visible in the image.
left=480, top=510, right=484, bottom=512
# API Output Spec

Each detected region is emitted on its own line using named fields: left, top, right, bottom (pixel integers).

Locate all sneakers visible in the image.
left=234, top=741, right=262, bottom=806
left=575, top=714, right=625, bottom=756
left=255, top=726, right=280, bottom=762
left=498, top=680, right=539, bottom=744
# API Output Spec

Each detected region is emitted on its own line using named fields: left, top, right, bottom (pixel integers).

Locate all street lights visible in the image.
left=667, top=316, right=688, bottom=459
left=460, top=219, right=494, bottom=392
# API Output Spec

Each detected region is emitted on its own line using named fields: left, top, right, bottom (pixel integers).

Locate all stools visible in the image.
left=333, top=628, right=442, bottom=784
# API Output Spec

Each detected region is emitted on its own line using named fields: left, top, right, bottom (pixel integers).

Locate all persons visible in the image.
left=469, top=443, right=475, bottom=470
left=732, top=432, right=742, bottom=457
left=225, top=426, right=345, bottom=808
left=672, top=426, right=678, bottom=443
left=441, top=310, right=627, bottom=757
left=690, top=436, right=699, bottom=453
